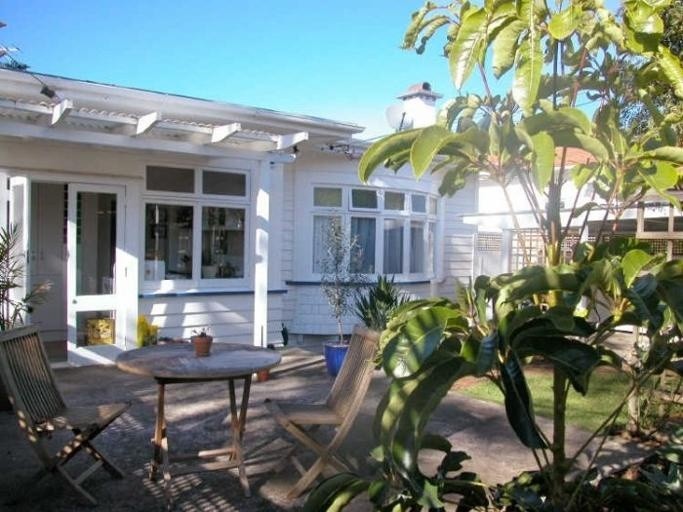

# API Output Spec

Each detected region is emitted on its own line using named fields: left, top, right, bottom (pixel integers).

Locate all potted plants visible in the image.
left=0, top=221, right=56, bottom=412
left=189, top=326, right=214, bottom=355
left=315, top=207, right=373, bottom=376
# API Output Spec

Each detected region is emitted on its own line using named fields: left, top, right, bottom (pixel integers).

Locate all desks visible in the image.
left=115, top=341, right=280, bottom=511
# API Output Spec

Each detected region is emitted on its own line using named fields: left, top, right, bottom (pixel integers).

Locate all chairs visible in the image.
left=263, top=324, right=388, bottom=501
left=0, top=323, right=131, bottom=505
